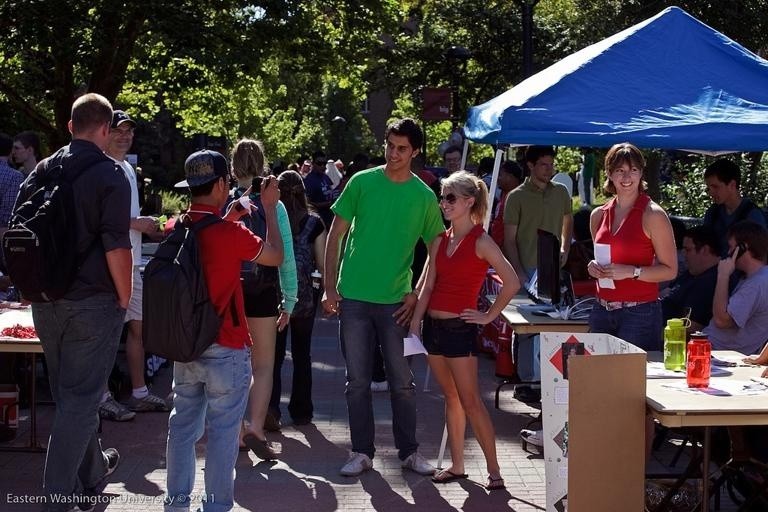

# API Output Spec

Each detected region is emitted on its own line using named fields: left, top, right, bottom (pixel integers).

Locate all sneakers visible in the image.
left=97, top=396, right=136, bottom=421
left=100, top=448, right=120, bottom=479
left=127, top=394, right=170, bottom=412
left=67, top=498, right=95, bottom=512
left=401, top=452, right=436, bottom=474
left=520, top=429, right=543, bottom=446
left=340, top=452, right=373, bottom=475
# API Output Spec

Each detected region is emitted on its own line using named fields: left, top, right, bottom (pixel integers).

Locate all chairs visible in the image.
left=669, top=341, right=766, bottom=468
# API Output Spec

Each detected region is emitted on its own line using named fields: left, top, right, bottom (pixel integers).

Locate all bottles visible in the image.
left=311, top=269, right=322, bottom=289
left=687, top=332, right=711, bottom=388
left=664, top=318, right=691, bottom=371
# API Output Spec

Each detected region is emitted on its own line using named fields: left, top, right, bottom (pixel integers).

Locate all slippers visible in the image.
left=486, top=476, right=504, bottom=490
left=238, top=417, right=281, bottom=459
left=432, top=470, right=468, bottom=482
left=293, top=420, right=310, bottom=425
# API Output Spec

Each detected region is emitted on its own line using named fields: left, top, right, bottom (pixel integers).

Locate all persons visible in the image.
left=1, top=92, right=768, bottom=512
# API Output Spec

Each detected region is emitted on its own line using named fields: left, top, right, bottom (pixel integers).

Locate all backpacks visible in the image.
left=1, top=154, right=116, bottom=304
left=142, top=216, right=221, bottom=364
left=289, top=214, right=317, bottom=319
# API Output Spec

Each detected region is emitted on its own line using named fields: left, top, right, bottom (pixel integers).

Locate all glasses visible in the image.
left=314, top=160, right=327, bottom=166
left=438, top=193, right=463, bottom=204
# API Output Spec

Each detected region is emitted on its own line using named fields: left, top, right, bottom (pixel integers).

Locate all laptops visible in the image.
left=561, top=268, right=593, bottom=313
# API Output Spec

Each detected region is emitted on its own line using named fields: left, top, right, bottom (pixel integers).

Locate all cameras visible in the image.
left=252, top=176, right=270, bottom=194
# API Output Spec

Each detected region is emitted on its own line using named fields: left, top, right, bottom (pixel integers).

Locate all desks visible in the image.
left=0, top=304, right=103, bottom=453
left=485, top=294, right=592, bottom=451
left=634, top=349, right=768, bottom=512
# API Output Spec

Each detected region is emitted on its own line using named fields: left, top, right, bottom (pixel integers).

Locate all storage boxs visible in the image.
left=0, top=384, right=20, bottom=429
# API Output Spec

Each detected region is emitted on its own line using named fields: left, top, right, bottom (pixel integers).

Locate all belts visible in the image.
left=595, top=297, right=646, bottom=311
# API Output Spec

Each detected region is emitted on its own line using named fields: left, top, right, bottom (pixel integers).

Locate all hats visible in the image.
left=174, top=149, right=228, bottom=188
left=111, top=110, right=138, bottom=129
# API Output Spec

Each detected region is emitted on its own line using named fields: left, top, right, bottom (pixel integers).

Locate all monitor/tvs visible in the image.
left=530, top=228, right=560, bottom=317
